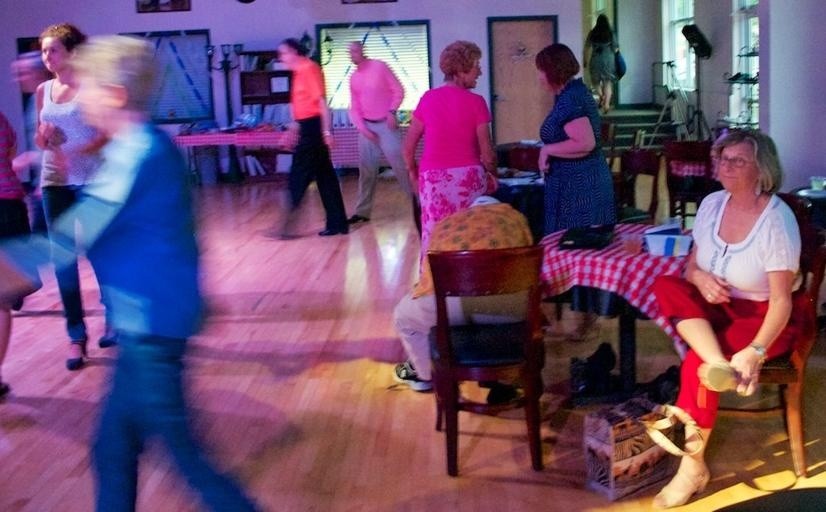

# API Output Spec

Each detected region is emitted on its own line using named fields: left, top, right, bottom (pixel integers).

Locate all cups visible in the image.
left=809, top=176, right=826, bottom=192
left=622, top=233, right=644, bottom=254
left=238, top=55, right=259, bottom=73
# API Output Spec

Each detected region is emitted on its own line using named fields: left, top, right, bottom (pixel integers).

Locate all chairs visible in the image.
left=613, top=145, right=640, bottom=202
left=428, top=242, right=547, bottom=477
left=718, top=223, right=826, bottom=480
left=496, top=142, right=542, bottom=170
left=601, top=123, right=616, bottom=170
left=617, top=149, right=660, bottom=223
left=777, top=192, right=813, bottom=223
left=665, top=141, right=722, bottom=229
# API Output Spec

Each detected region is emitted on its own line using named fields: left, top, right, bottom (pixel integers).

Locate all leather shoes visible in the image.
left=652, top=463, right=710, bottom=510
left=696, top=361, right=755, bottom=397
left=347, top=215, right=369, bottom=223
left=319, top=229, right=348, bottom=236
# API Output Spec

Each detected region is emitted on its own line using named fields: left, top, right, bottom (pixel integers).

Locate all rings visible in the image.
left=707, top=294, right=712, bottom=299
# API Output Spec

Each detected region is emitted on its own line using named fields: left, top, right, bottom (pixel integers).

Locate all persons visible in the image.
left=584, top=14, right=619, bottom=114
left=652, top=128, right=803, bottom=509
left=399, top=41, right=499, bottom=282
left=391, top=193, right=534, bottom=405
left=535, top=43, right=618, bottom=236
left=11, top=49, right=54, bottom=266
left=32, top=22, right=121, bottom=372
left=346, top=41, right=413, bottom=225
left=0, top=35, right=260, bottom=511
left=0, top=110, right=32, bottom=398
left=255, top=38, right=349, bottom=240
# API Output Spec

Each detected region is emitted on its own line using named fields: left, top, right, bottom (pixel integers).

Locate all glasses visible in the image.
left=719, top=156, right=756, bottom=168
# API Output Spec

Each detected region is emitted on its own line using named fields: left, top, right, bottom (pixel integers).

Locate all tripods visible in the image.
left=648, top=66, right=690, bottom=152
left=687, top=57, right=712, bottom=141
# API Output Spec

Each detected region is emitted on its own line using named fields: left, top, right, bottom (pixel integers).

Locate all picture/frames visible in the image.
left=136, top=0, right=190, bottom=13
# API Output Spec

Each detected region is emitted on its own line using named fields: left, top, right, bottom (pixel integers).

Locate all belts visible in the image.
left=363, top=118, right=387, bottom=123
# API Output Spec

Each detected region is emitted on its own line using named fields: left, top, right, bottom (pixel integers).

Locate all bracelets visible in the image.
left=322, top=129, right=334, bottom=137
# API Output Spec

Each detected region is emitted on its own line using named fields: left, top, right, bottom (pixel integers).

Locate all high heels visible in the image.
left=67, top=338, right=86, bottom=370
left=99, top=333, right=118, bottom=347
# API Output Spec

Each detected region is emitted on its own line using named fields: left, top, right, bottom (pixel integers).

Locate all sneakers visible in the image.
left=486, top=390, right=516, bottom=403
left=393, top=361, right=432, bottom=392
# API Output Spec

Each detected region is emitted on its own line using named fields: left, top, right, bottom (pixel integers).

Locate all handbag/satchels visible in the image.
left=583, top=393, right=675, bottom=501
left=559, top=222, right=615, bottom=250
left=616, top=50, right=627, bottom=78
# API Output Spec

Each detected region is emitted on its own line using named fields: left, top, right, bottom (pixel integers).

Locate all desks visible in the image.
left=671, top=159, right=721, bottom=182
left=175, top=127, right=423, bottom=187
left=541, top=224, right=693, bottom=401
left=494, top=176, right=544, bottom=238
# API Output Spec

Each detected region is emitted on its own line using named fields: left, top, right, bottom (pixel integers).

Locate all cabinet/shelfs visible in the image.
left=715, top=45, right=759, bottom=129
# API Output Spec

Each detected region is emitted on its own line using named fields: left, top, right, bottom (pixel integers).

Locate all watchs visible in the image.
left=750, top=343, right=767, bottom=358
left=389, top=110, right=396, bottom=115
